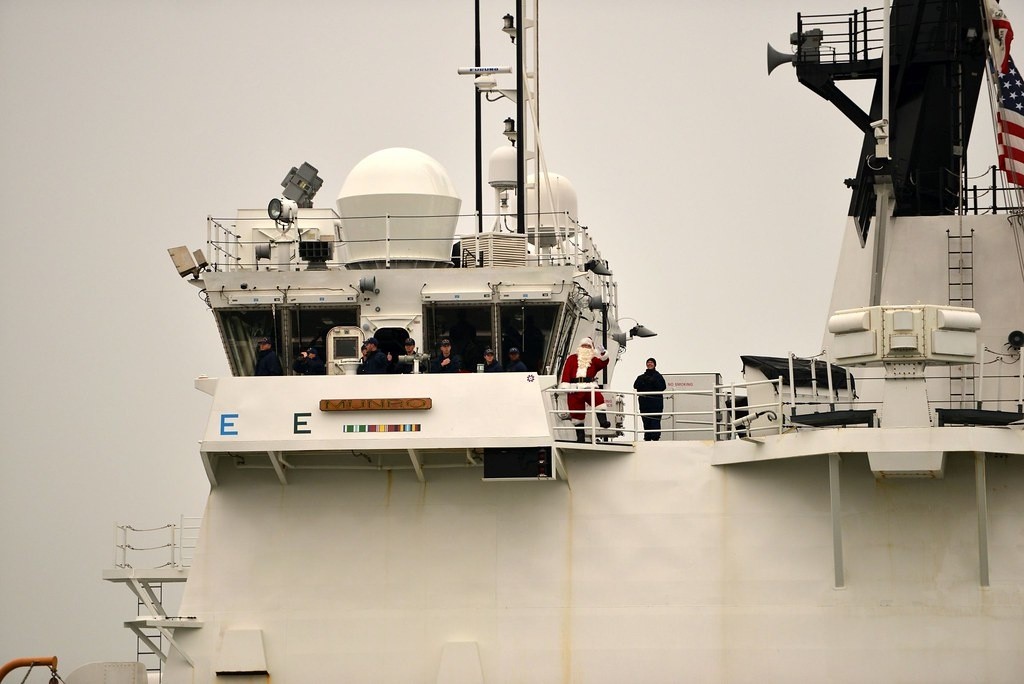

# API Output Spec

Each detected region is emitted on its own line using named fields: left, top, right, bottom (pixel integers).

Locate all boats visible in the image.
left=1, top=3, right=1024, bottom=679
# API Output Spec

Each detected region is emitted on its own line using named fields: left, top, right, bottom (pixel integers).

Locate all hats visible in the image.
left=306, top=348, right=318, bottom=355
left=405, top=338, right=415, bottom=345
left=646, top=358, right=656, bottom=365
left=440, top=339, right=451, bottom=347
left=257, top=337, right=272, bottom=345
left=363, top=338, right=378, bottom=345
left=580, top=337, right=594, bottom=349
left=508, top=348, right=520, bottom=354
left=484, top=349, right=494, bottom=355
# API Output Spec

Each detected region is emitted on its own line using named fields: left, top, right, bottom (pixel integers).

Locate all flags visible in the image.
left=983, top=0, right=1024, bottom=188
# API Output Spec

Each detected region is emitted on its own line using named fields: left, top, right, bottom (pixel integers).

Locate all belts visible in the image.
left=570, top=377, right=591, bottom=383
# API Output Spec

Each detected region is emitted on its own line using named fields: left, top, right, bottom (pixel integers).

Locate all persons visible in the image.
left=561, top=336, right=611, bottom=443
left=254, top=335, right=282, bottom=376
left=292, top=347, right=325, bottom=375
left=362, top=337, right=388, bottom=374
left=357, top=344, right=369, bottom=374
left=387, top=338, right=427, bottom=374
left=472, top=348, right=503, bottom=373
left=433, top=339, right=461, bottom=373
left=634, top=358, right=667, bottom=441
left=503, top=348, right=527, bottom=372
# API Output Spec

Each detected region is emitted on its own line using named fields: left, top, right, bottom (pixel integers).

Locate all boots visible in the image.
left=575, top=423, right=585, bottom=443
left=596, top=410, right=610, bottom=427
